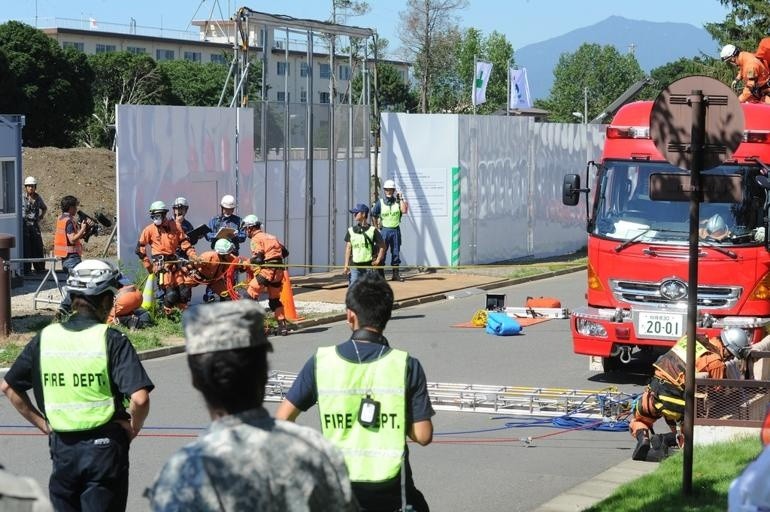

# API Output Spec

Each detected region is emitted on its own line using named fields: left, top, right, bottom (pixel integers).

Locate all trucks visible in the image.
left=561, top=97, right=770, bottom=382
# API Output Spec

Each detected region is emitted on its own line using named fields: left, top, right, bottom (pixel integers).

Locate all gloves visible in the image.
left=753, top=227, right=765, bottom=242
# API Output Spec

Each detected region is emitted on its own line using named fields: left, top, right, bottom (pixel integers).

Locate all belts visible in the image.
left=127, top=286, right=142, bottom=292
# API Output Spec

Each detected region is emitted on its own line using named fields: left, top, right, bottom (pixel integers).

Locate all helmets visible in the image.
left=720, top=44, right=740, bottom=62
left=706, top=214, right=725, bottom=235
left=149, top=195, right=262, bottom=255
left=65, top=259, right=124, bottom=296
left=383, top=180, right=395, bottom=189
left=721, top=328, right=748, bottom=360
left=24, top=176, right=38, bottom=186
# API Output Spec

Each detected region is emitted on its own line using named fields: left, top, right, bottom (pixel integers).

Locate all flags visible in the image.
left=510, top=67, right=533, bottom=110
left=471, top=60, right=493, bottom=106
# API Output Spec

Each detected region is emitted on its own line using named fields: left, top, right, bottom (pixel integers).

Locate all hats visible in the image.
left=349, top=204, right=369, bottom=213
left=182, top=298, right=273, bottom=355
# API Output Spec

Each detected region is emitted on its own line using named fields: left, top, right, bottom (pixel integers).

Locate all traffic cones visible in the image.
left=139, top=272, right=161, bottom=315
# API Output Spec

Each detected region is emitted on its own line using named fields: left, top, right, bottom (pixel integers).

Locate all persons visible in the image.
left=1, top=257, right=157, bottom=512
left=141, top=296, right=359, bottom=512
left=720, top=43, right=770, bottom=104
left=341, top=202, right=388, bottom=289
left=103, top=194, right=290, bottom=333
left=52, top=195, right=95, bottom=317
left=368, top=178, right=410, bottom=283
left=628, top=323, right=752, bottom=461
left=276, top=269, right=437, bottom=512
left=21, top=175, right=49, bottom=277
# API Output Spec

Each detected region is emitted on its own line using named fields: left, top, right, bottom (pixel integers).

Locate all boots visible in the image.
left=392, top=265, right=404, bottom=282
left=633, top=429, right=650, bottom=461
left=275, top=319, right=288, bottom=336
